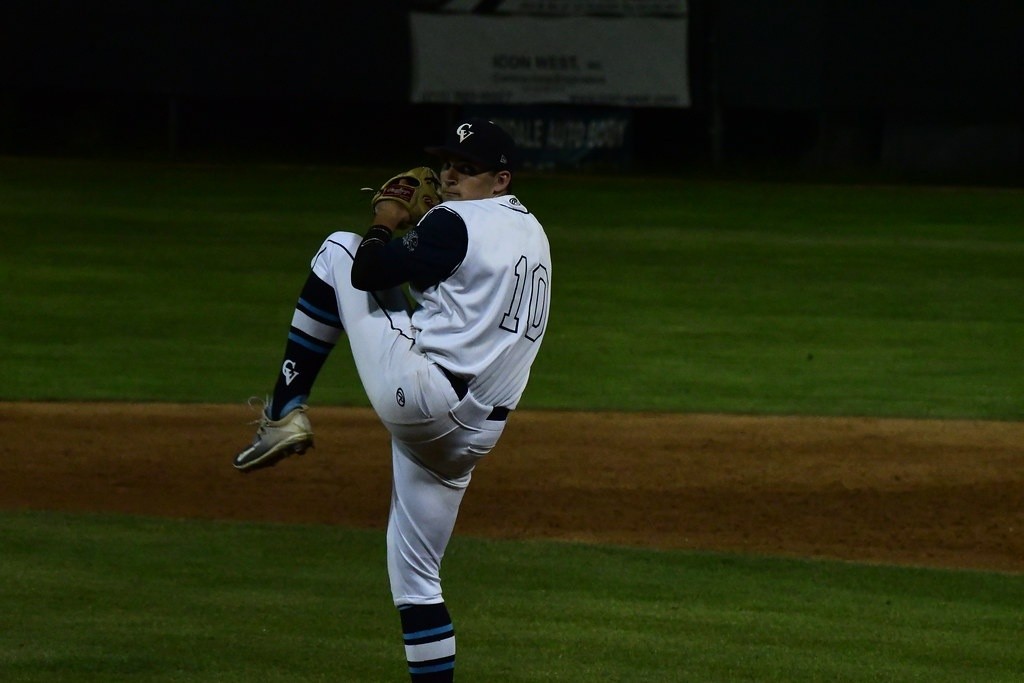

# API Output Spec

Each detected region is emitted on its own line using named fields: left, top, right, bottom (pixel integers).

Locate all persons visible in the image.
left=232, top=113, right=553, bottom=683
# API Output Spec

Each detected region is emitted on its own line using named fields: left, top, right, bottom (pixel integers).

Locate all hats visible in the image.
left=426, top=117, right=516, bottom=170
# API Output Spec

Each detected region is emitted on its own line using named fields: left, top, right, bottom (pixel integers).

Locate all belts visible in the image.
left=438, top=364, right=510, bottom=422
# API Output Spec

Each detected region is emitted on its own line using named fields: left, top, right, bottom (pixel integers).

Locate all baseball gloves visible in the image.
left=362, top=167, right=442, bottom=229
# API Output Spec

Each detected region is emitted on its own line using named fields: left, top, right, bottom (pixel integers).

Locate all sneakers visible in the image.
left=231, top=405, right=315, bottom=472
